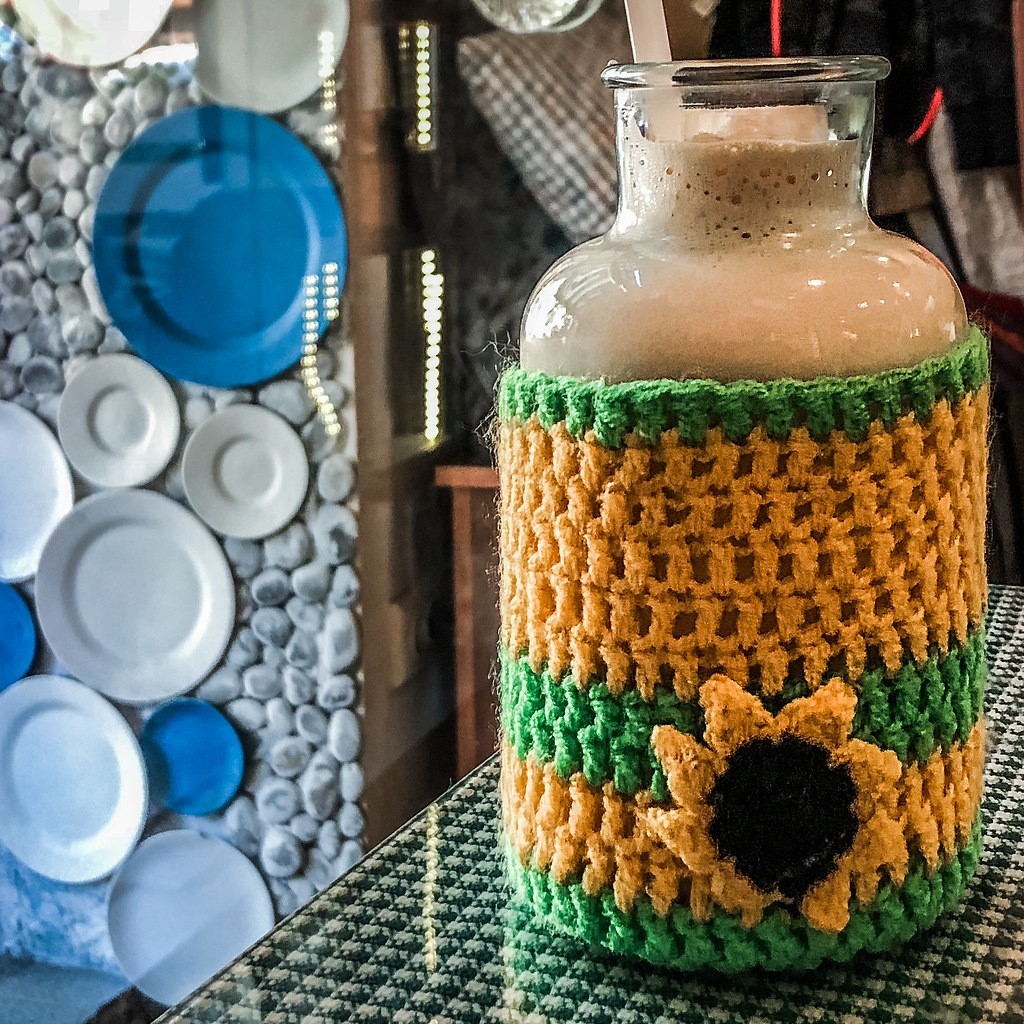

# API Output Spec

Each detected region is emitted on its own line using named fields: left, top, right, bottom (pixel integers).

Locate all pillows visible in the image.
left=457, top=9, right=648, bottom=242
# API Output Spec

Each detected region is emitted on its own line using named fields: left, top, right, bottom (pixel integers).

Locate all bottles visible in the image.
left=519, top=59, right=961, bottom=380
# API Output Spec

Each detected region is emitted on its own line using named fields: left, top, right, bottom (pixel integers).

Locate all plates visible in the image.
left=57, top=354, right=179, bottom=490
left=138, top=697, right=243, bottom=816
left=92, top=106, right=348, bottom=389
left=0, top=582, right=35, bottom=691
left=474, top=0, right=580, bottom=34
left=181, top=403, right=309, bottom=540
left=0, top=400, right=74, bottom=583
left=12, top=0, right=173, bottom=66
left=0, top=676, right=150, bottom=884
left=104, top=828, right=276, bottom=1007
left=545, top=0, right=604, bottom=32
left=185, top=0, right=351, bottom=116
left=35, top=489, right=235, bottom=707
